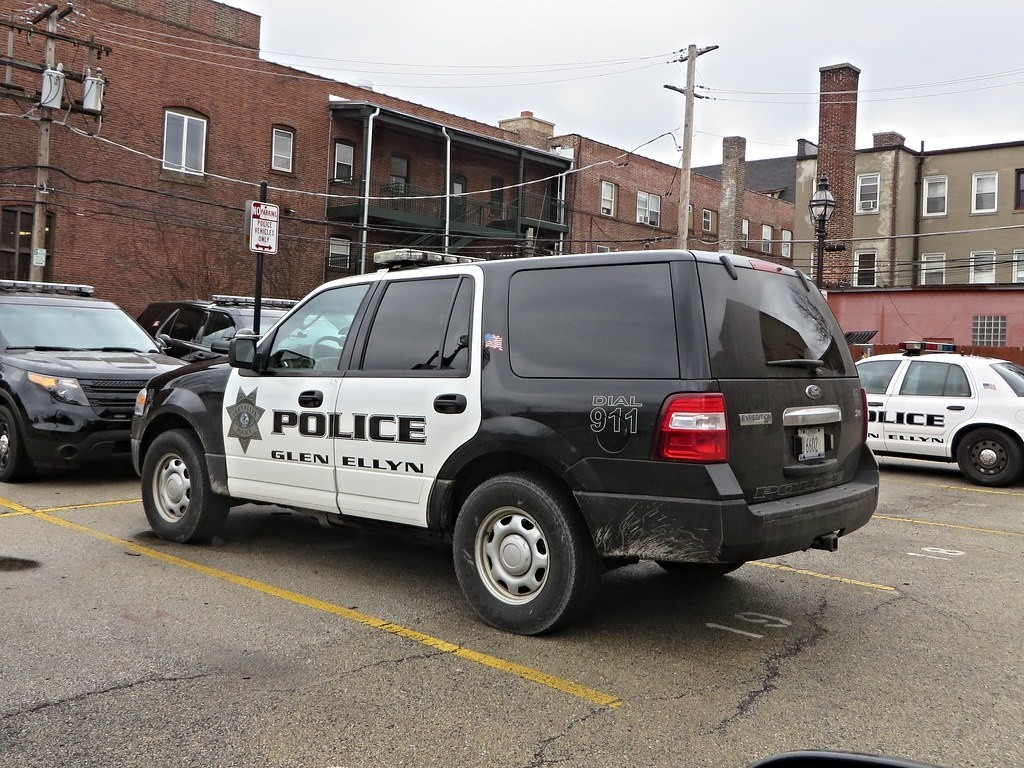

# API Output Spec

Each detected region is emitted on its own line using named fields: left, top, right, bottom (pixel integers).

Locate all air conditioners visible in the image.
left=860, top=200, right=877, bottom=210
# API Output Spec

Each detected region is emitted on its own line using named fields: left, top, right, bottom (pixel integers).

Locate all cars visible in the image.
left=854, top=339, right=1024, bottom=482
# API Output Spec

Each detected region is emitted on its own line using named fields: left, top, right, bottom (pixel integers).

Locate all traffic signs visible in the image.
left=250, top=201, right=281, bottom=256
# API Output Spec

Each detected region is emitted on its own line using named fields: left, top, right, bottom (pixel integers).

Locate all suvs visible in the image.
left=127, top=240, right=882, bottom=636
left=134, top=293, right=344, bottom=366
left=0, top=278, right=197, bottom=480
